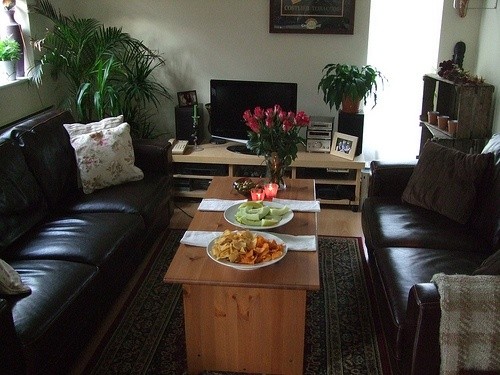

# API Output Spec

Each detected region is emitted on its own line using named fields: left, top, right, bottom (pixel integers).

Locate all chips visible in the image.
left=212, top=229, right=285, bottom=264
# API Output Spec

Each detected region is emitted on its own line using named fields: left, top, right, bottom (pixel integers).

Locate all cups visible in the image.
left=437, top=115, right=449, bottom=130
left=448, top=120, right=457, bottom=135
left=250, top=188, right=265, bottom=201
left=427, top=111, right=439, bottom=124
left=264, top=184, right=278, bottom=199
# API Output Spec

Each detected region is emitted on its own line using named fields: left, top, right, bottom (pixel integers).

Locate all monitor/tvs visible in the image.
left=209, top=79, right=297, bottom=156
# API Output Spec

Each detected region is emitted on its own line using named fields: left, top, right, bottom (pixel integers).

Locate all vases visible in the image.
left=261, top=152, right=286, bottom=191
left=4, top=9, right=25, bottom=79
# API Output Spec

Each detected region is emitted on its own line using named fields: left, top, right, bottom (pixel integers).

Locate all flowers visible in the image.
left=241, top=105, right=310, bottom=185
left=1, top=0, right=16, bottom=10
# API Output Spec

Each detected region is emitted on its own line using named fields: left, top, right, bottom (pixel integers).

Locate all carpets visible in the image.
left=78, top=226, right=393, bottom=375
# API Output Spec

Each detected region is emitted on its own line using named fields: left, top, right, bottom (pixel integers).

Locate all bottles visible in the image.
left=270, top=152, right=282, bottom=191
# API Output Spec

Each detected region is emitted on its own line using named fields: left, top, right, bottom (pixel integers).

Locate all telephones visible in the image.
left=168, top=138, right=188, bottom=154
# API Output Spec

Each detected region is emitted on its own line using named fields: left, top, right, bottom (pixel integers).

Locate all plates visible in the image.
left=224, top=201, right=294, bottom=230
left=206, top=231, right=287, bottom=271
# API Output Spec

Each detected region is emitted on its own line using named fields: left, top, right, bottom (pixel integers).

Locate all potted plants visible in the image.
left=0, top=37, right=23, bottom=80
left=317, top=62, right=387, bottom=114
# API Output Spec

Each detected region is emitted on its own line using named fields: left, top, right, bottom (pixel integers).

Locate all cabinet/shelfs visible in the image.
left=166, top=135, right=365, bottom=212
left=418, top=74, right=494, bottom=153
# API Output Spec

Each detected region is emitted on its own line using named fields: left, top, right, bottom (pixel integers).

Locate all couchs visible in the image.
left=362, top=133, right=500, bottom=375
left=0, top=104, right=174, bottom=375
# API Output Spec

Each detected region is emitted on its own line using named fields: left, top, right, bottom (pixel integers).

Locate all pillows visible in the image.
left=62, top=115, right=145, bottom=194
left=0, top=259, right=30, bottom=295
left=401, top=137, right=494, bottom=225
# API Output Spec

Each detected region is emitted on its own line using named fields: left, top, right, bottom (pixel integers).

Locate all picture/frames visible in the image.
left=177, top=90, right=198, bottom=107
left=329, top=131, right=358, bottom=160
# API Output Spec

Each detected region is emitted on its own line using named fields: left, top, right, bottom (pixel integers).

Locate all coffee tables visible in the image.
left=161, top=176, right=320, bottom=374
